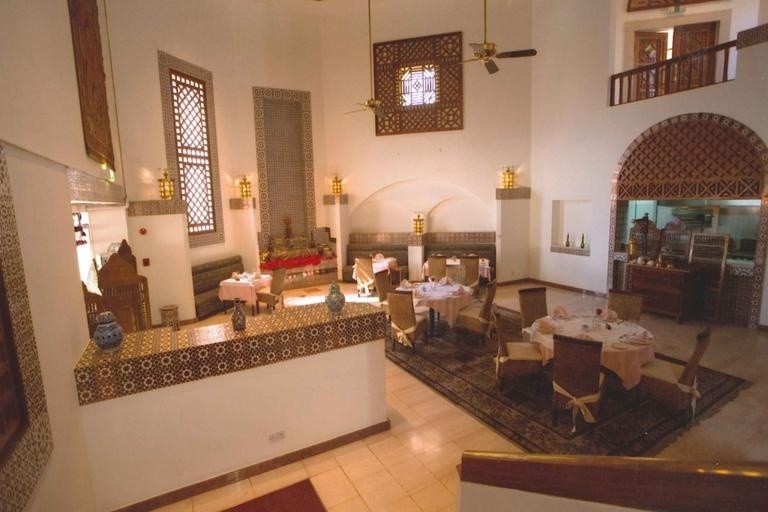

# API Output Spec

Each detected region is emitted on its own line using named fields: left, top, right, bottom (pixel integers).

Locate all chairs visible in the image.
left=373, top=256, right=498, bottom=356
left=255, top=268, right=286, bottom=314
left=491, top=285, right=712, bottom=435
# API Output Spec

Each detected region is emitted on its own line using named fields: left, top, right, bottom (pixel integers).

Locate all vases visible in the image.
left=325, top=279, right=345, bottom=316
left=93, top=311, right=124, bottom=353
left=231, top=297, right=246, bottom=331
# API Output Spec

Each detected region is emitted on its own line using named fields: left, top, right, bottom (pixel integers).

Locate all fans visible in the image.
left=344, top=1, right=402, bottom=118
left=455, top=0, right=536, bottom=75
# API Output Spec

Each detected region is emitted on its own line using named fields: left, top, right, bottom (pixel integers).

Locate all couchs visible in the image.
left=192, top=256, right=244, bottom=321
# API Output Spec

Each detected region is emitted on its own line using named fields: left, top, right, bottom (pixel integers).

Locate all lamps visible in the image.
left=239, top=176, right=252, bottom=198
left=332, top=177, right=341, bottom=195
left=502, top=168, right=515, bottom=189
left=413, top=214, right=424, bottom=233
left=158, top=168, right=175, bottom=200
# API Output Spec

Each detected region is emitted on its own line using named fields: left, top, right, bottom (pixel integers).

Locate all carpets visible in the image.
left=383, top=300, right=756, bottom=455
left=219, top=479, right=328, bottom=512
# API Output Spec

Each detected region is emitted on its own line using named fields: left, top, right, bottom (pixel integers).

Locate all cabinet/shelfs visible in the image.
left=626, top=260, right=708, bottom=324
